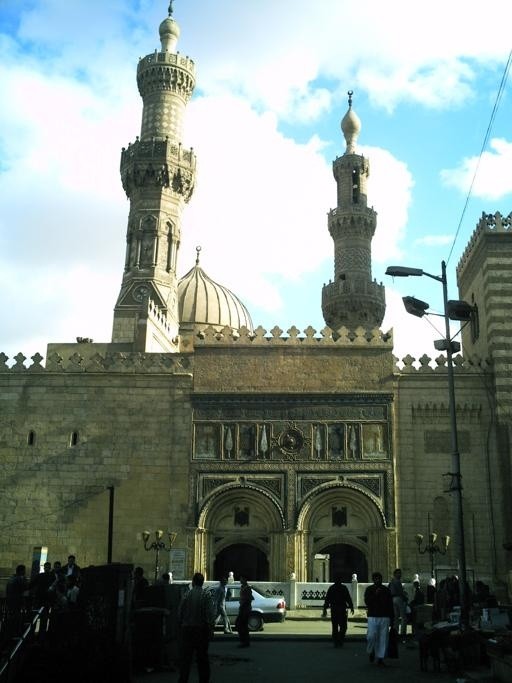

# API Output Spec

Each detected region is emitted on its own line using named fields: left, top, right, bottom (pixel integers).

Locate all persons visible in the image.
left=364, top=573, right=392, bottom=669
left=406, top=576, right=491, bottom=623
left=176, top=572, right=216, bottom=683
left=6, top=555, right=81, bottom=610
left=132, top=567, right=169, bottom=608
left=213, top=576, right=233, bottom=634
left=390, top=569, right=408, bottom=645
left=322, top=575, right=354, bottom=648
left=235, top=574, right=254, bottom=649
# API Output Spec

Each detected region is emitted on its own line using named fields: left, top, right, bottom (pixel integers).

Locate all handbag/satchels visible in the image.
left=387, top=623, right=399, bottom=659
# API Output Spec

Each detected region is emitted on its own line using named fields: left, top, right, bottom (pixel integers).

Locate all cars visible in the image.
left=214, top=585, right=287, bottom=631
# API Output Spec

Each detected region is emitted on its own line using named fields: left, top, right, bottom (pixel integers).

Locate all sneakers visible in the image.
left=368, top=653, right=386, bottom=668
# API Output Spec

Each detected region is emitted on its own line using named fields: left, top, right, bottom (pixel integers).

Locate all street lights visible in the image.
left=141, top=530, right=178, bottom=584
left=385, top=262, right=474, bottom=629
